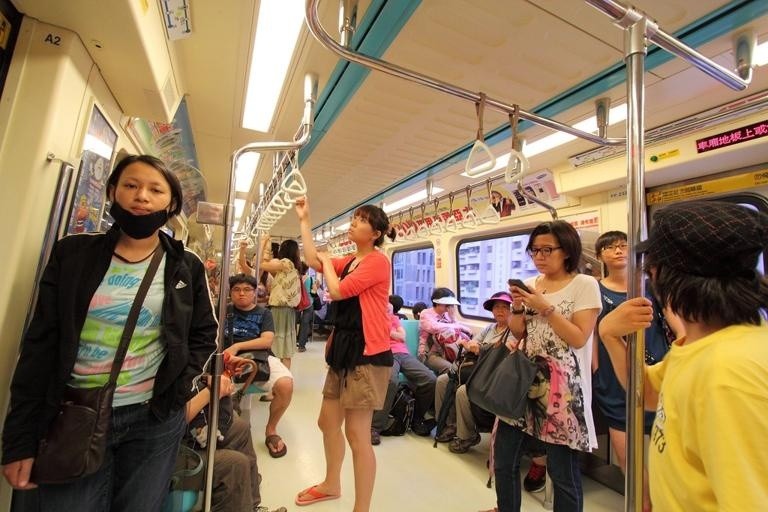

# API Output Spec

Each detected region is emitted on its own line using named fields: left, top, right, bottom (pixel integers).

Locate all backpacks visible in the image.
left=433, top=323, right=474, bottom=361
left=381, top=382, right=415, bottom=437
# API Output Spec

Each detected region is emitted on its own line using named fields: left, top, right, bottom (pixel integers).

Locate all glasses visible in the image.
left=599, top=242, right=626, bottom=253
left=526, top=244, right=560, bottom=258
left=231, top=286, right=255, bottom=294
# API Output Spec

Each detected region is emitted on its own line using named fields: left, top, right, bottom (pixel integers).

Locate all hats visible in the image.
left=633, top=198, right=768, bottom=279
left=482, top=293, right=514, bottom=311
left=432, top=295, right=461, bottom=307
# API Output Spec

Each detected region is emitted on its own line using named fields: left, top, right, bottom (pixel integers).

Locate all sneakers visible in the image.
left=522, top=460, right=548, bottom=495
left=449, top=435, right=482, bottom=453
left=371, top=430, right=380, bottom=446
left=433, top=424, right=455, bottom=442
left=254, top=504, right=287, bottom=512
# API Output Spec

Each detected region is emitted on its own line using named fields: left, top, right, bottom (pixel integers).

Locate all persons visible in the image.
left=296, top=195, right=396, bottom=512
left=491, top=191, right=516, bottom=217
left=205, top=241, right=328, bottom=459
left=0, top=155, right=220, bottom=512
left=172, top=372, right=287, bottom=512
left=478, top=220, right=604, bottom=512
left=590, top=231, right=670, bottom=511
left=369, top=285, right=549, bottom=495
left=597, top=200, right=768, bottom=511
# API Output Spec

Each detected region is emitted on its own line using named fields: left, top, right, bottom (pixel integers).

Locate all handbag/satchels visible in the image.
left=465, top=315, right=535, bottom=421
left=190, top=374, right=234, bottom=450
left=295, top=277, right=311, bottom=311
left=309, top=277, right=322, bottom=312
left=27, top=377, right=120, bottom=486
left=232, top=349, right=270, bottom=383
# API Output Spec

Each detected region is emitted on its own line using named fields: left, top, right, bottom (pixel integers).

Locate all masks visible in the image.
left=109, top=186, right=173, bottom=241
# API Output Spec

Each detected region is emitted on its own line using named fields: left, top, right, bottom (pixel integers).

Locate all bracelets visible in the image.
left=540, top=305, right=555, bottom=318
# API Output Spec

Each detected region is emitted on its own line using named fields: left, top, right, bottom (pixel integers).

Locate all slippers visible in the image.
left=264, top=434, right=287, bottom=459
left=296, top=483, right=341, bottom=506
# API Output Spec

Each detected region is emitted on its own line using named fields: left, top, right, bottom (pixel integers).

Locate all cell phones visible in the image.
left=508, top=279, right=530, bottom=293
